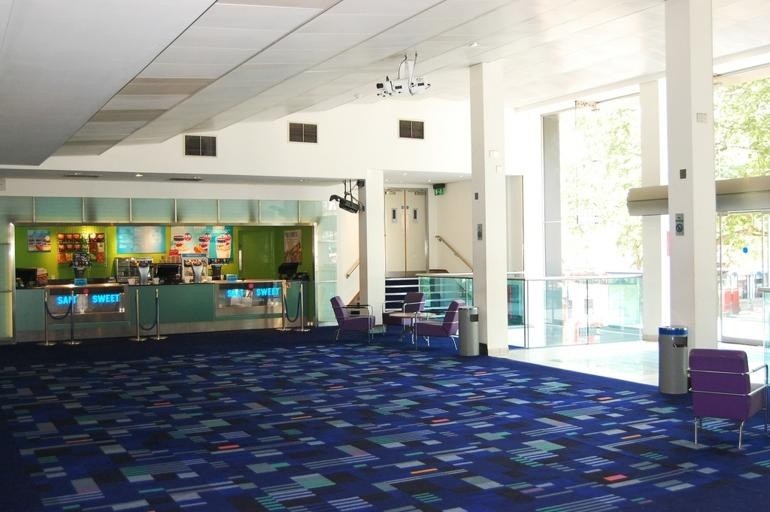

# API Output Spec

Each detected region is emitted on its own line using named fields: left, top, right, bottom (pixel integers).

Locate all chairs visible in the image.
left=330, top=295, right=376, bottom=345
left=395, top=292, right=465, bottom=352
left=686, top=348, right=768, bottom=450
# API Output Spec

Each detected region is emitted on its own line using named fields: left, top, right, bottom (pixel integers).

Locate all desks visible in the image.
left=389, top=311, right=437, bottom=345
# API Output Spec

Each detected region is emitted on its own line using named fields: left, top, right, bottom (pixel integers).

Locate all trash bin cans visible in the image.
left=659, top=327, right=689, bottom=394
left=458, top=306, right=480, bottom=356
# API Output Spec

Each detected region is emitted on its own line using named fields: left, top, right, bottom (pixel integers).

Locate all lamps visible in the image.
left=330, top=180, right=364, bottom=215
